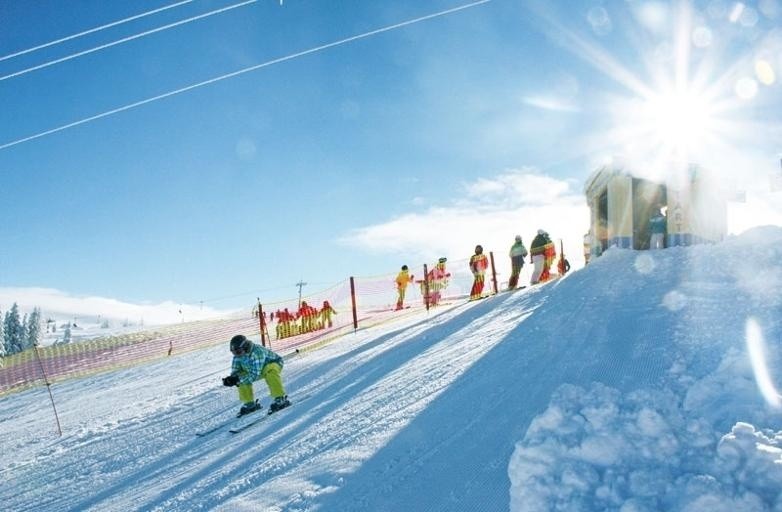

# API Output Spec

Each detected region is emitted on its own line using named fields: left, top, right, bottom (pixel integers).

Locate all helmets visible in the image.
left=439, top=257, right=447, bottom=263
left=475, top=245, right=483, bottom=255
left=229, top=335, right=252, bottom=357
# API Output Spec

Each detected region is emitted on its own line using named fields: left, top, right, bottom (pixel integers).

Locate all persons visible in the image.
left=394, top=266, right=414, bottom=310
left=557, top=254, right=571, bottom=274
left=222, top=335, right=287, bottom=411
left=584, top=228, right=592, bottom=265
left=423, top=257, right=451, bottom=307
left=270, top=301, right=337, bottom=341
left=507, top=236, right=528, bottom=289
left=530, top=229, right=547, bottom=284
left=468, top=245, right=489, bottom=300
left=646, top=204, right=703, bottom=248
left=543, top=233, right=556, bottom=280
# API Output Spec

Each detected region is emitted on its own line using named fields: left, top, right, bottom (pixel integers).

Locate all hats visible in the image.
left=538, top=228, right=546, bottom=235
left=514, top=234, right=522, bottom=243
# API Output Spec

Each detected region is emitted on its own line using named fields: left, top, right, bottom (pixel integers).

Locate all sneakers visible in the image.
left=267, top=396, right=291, bottom=415
left=235, top=401, right=263, bottom=419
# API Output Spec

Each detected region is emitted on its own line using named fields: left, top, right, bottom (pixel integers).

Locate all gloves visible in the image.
left=223, top=375, right=241, bottom=388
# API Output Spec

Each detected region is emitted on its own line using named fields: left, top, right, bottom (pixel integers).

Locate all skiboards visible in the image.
left=196, top=394, right=311, bottom=436
